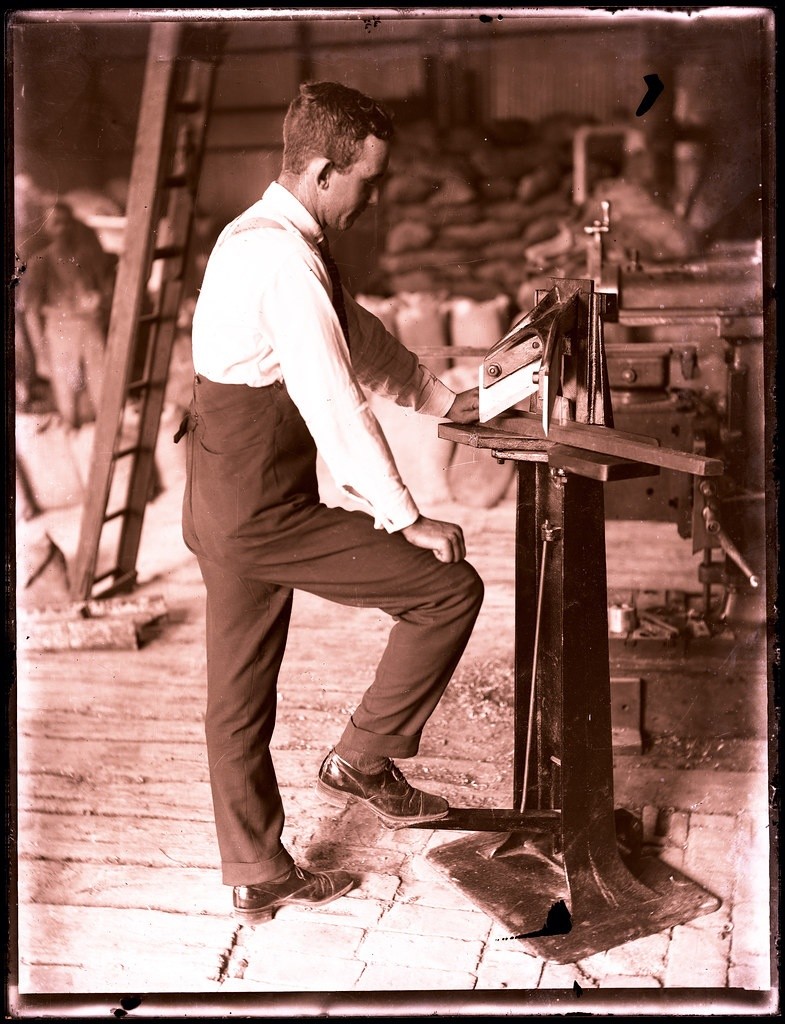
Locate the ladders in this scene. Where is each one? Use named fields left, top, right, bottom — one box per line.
left=66, top=21, right=234, bottom=602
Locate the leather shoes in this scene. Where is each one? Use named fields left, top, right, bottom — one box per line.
left=316, top=746, right=450, bottom=823
left=232, top=864, right=355, bottom=924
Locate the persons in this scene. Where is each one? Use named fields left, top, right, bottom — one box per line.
left=21, top=202, right=114, bottom=437
left=173, top=81, right=511, bottom=925
